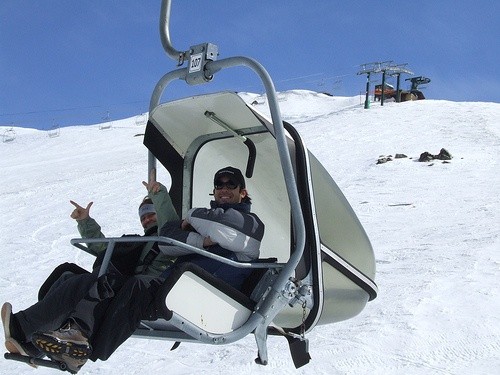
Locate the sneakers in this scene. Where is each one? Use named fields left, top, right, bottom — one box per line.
left=5, top=337, right=45, bottom=361
left=1, top=303, right=25, bottom=344
left=31, top=328, right=94, bottom=359
left=47, top=350, right=88, bottom=375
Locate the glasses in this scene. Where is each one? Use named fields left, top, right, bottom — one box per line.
left=213, top=180, right=240, bottom=190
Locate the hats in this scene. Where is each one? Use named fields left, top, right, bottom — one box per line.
left=214, top=166, right=245, bottom=190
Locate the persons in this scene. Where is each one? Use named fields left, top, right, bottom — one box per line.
left=33, top=167, right=264, bottom=375
left=1, top=169, right=182, bottom=360
